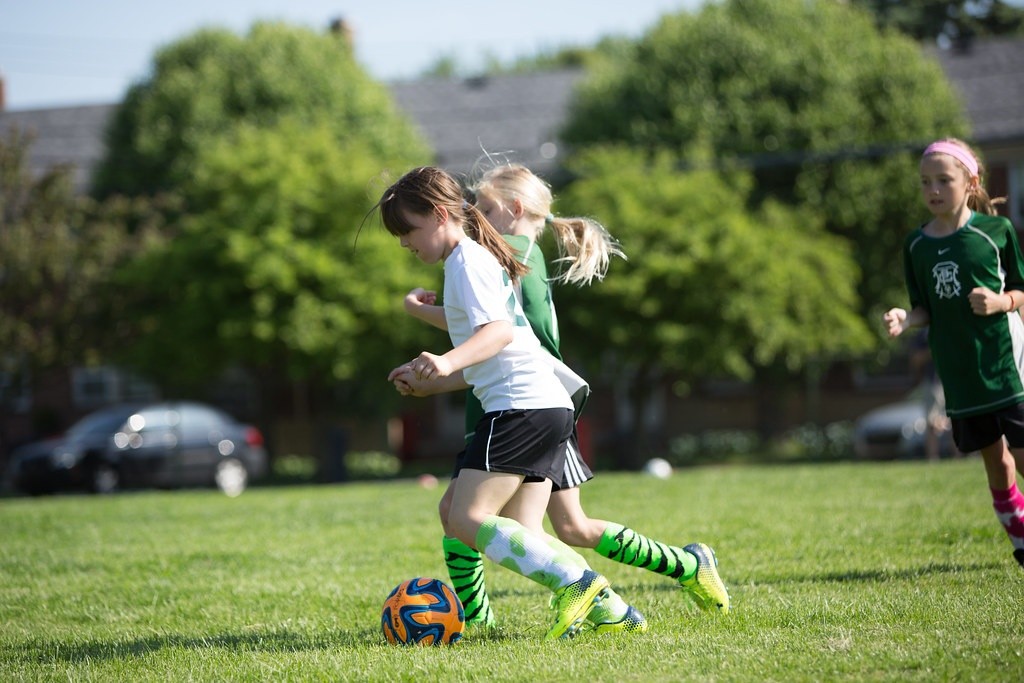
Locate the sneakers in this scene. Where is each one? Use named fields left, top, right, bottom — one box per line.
left=462, top=619, right=499, bottom=638
left=543, top=570, right=610, bottom=640
left=583, top=606, right=647, bottom=641
left=677, top=543, right=732, bottom=619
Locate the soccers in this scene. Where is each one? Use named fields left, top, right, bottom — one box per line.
left=381, top=576, right=466, bottom=647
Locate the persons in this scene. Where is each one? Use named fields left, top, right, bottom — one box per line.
left=882, top=138, right=1024, bottom=569
left=403, top=168, right=731, bottom=637
left=373, top=165, right=611, bottom=642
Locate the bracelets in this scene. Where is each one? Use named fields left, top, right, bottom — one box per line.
left=1004, top=291, right=1015, bottom=312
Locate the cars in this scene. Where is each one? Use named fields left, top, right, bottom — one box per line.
left=0, top=400, right=267, bottom=497
left=850, top=377, right=959, bottom=460
